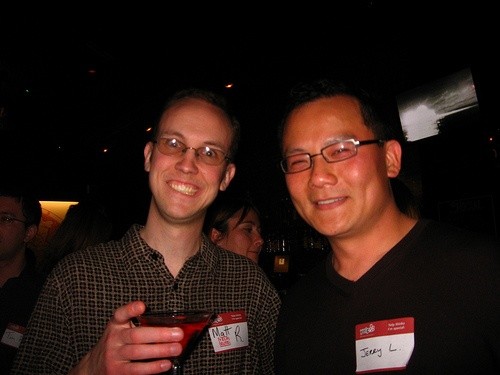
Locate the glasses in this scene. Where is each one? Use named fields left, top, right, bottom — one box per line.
left=155, top=136, right=229, bottom=166
left=0, top=216, right=24, bottom=225
left=281, top=139, right=377, bottom=174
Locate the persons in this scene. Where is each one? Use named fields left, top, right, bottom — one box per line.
left=0, top=177, right=119, bottom=375
left=11, top=88, right=282, bottom=375
left=275, top=74, right=500, bottom=375
left=202, top=195, right=264, bottom=264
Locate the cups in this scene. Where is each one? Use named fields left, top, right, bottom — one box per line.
left=136, top=311, right=215, bottom=375
